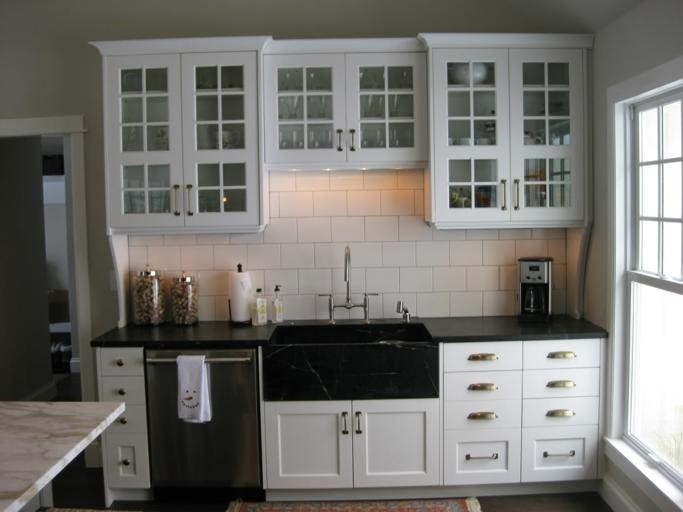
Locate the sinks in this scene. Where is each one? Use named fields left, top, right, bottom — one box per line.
left=266, top=325, right=435, bottom=344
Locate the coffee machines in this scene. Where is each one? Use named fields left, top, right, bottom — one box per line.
left=517, top=256, right=554, bottom=325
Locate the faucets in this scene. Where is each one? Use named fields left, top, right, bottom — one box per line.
left=340, top=246, right=356, bottom=323
left=396, top=300, right=409, bottom=324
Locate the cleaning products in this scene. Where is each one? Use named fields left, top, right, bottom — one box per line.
left=251, top=288, right=267, bottom=327
left=269, top=284, right=285, bottom=324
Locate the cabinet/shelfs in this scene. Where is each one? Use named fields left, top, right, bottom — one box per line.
left=440, top=341, right=521, bottom=500
left=415, top=31, right=595, bottom=233
left=522, top=336, right=607, bottom=497
left=93, top=347, right=151, bottom=509
left=86, top=33, right=273, bottom=235
left=261, top=398, right=440, bottom=504
left=262, top=36, right=428, bottom=175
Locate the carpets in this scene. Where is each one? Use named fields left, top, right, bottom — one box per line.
left=224, top=495, right=481, bottom=512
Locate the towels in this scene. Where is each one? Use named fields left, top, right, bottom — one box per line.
left=175, top=355, right=214, bottom=425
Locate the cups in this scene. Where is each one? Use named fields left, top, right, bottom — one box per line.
left=358, top=66, right=414, bottom=147
left=277, top=67, right=333, bottom=149
left=122, top=177, right=166, bottom=212
left=476, top=138, right=490, bottom=145
left=447, top=137, right=453, bottom=146
left=459, top=137, right=470, bottom=144
left=198, top=195, right=208, bottom=211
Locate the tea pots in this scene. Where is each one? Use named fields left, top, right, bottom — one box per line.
left=523, top=129, right=543, bottom=144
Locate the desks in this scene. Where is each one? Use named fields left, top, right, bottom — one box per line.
left=0, top=400, right=130, bottom=512
